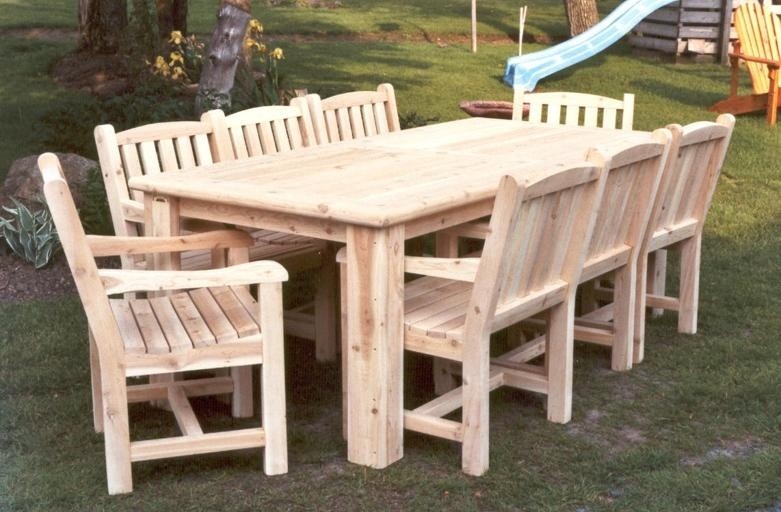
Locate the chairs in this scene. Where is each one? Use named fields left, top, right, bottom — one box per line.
left=531, top=113, right=734, bottom=367
left=335, top=150, right=609, bottom=477
left=94, top=118, right=338, bottom=408
left=432, top=127, right=670, bottom=413
left=436, top=91, right=637, bottom=259
left=204, top=96, right=347, bottom=328
left=37, top=150, right=290, bottom=494
left=710, top=2, right=781, bottom=127
left=304, top=82, right=428, bottom=280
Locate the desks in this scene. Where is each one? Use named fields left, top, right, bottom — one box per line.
left=127, top=116, right=659, bottom=468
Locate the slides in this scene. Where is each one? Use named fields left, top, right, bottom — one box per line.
left=504, top=0, right=676, bottom=93
